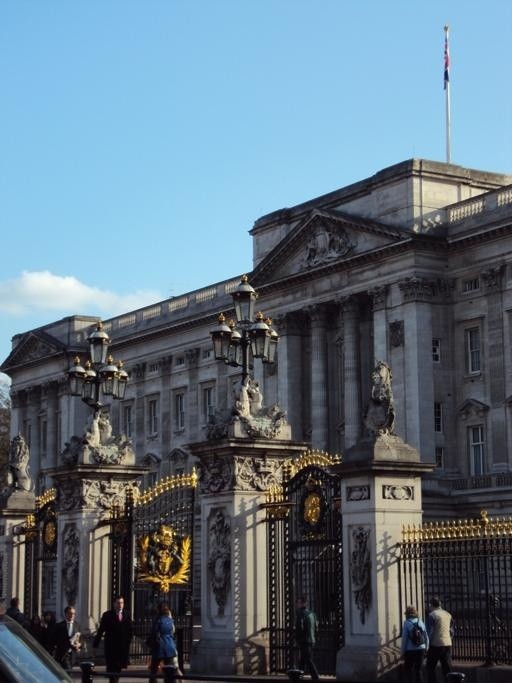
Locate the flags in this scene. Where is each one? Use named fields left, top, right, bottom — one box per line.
left=442, top=35, right=449, bottom=91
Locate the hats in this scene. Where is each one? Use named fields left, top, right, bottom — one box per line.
left=404, top=606, right=418, bottom=617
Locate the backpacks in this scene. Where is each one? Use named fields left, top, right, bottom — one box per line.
left=407, top=620, right=427, bottom=647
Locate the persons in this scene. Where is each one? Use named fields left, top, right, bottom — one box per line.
left=426, top=598, right=454, bottom=682
left=0, top=596, right=184, bottom=683
left=400, top=607, right=430, bottom=682
left=295, top=597, right=320, bottom=683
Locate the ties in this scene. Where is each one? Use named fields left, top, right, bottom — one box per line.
left=118, top=610, right=123, bottom=621
left=67, top=624, right=73, bottom=636
